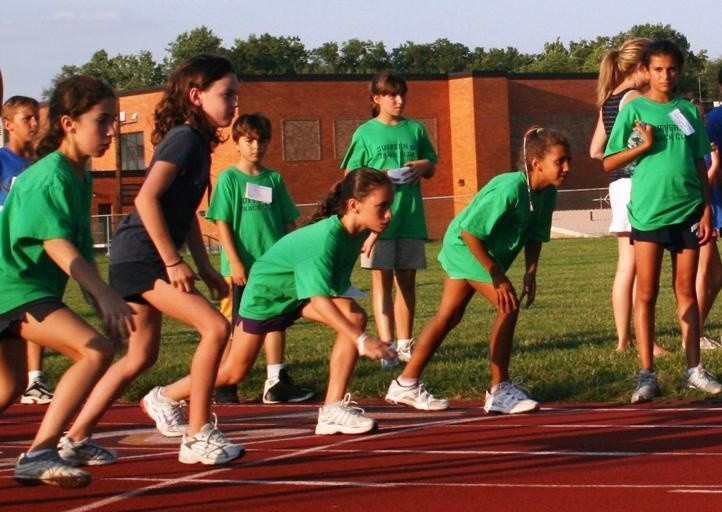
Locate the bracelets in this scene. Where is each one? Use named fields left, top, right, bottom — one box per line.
left=163, top=256, right=185, bottom=268
left=357, top=328, right=371, bottom=358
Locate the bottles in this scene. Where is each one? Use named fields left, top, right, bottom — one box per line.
left=621, top=120, right=646, bottom=175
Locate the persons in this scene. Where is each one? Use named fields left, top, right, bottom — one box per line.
left=3, top=92, right=56, bottom=405
left=204, top=111, right=316, bottom=405
left=138, top=165, right=396, bottom=438
left=598, top=40, right=721, bottom=406
left=678, top=103, right=721, bottom=351
left=337, top=68, right=442, bottom=373
left=3, top=74, right=140, bottom=491
left=587, top=33, right=676, bottom=361
left=54, top=50, right=249, bottom=467
left=382, top=121, right=573, bottom=420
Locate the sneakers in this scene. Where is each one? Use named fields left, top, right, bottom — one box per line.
left=384, top=379, right=449, bottom=411
left=681, top=362, right=721, bottom=395
left=395, top=337, right=416, bottom=362
left=140, top=386, right=188, bottom=438
left=178, top=412, right=241, bottom=466
left=13, top=447, right=91, bottom=488
left=213, top=384, right=240, bottom=405
left=681, top=336, right=720, bottom=350
left=315, top=393, right=378, bottom=435
left=56, top=436, right=117, bottom=467
left=263, top=368, right=315, bottom=404
left=483, top=376, right=539, bottom=414
left=20, top=375, right=54, bottom=405
left=631, top=369, right=662, bottom=403
left=380, top=342, right=400, bottom=370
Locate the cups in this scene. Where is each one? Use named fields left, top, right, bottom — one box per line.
left=387, top=167, right=410, bottom=186
